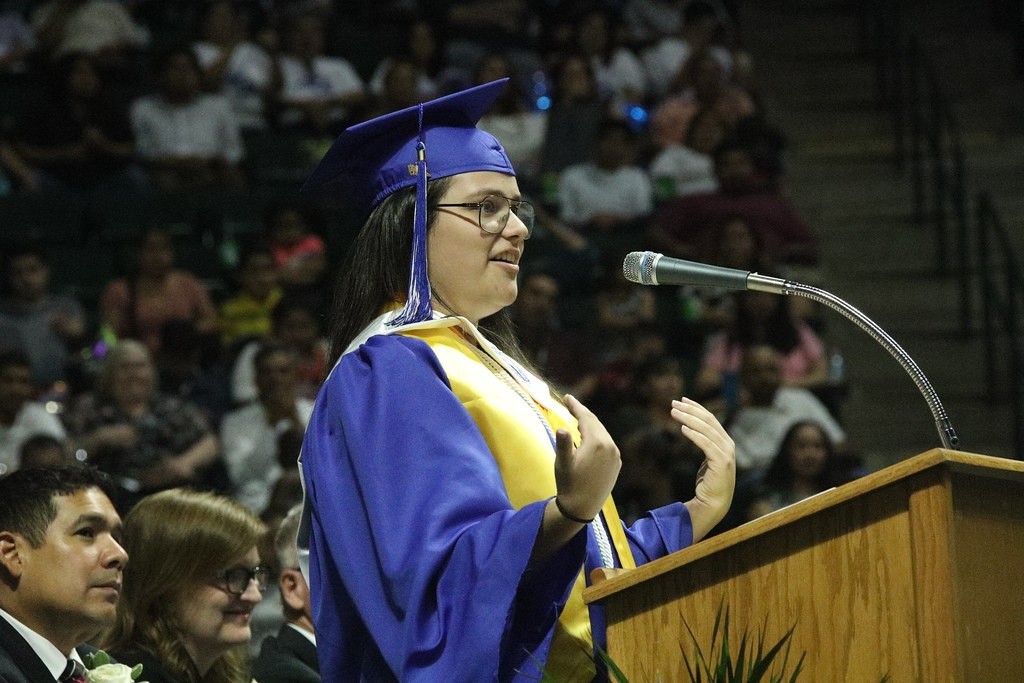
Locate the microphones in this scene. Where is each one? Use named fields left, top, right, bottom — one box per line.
left=623, top=250, right=961, bottom=453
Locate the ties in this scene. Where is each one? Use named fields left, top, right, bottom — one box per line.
left=60, top=659, right=86, bottom=683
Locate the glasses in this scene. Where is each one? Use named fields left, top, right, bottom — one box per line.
left=210, top=563, right=272, bottom=595
left=427, top=195, right=535, bottom=240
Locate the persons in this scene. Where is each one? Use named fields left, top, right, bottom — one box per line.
left=0, top=0, right=858, bottom=682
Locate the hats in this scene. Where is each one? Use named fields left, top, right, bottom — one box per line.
left=301, top=78, right=516, bottom=329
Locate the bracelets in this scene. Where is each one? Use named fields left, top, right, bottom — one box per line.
left=555, top=497, right=594, bottom=524
